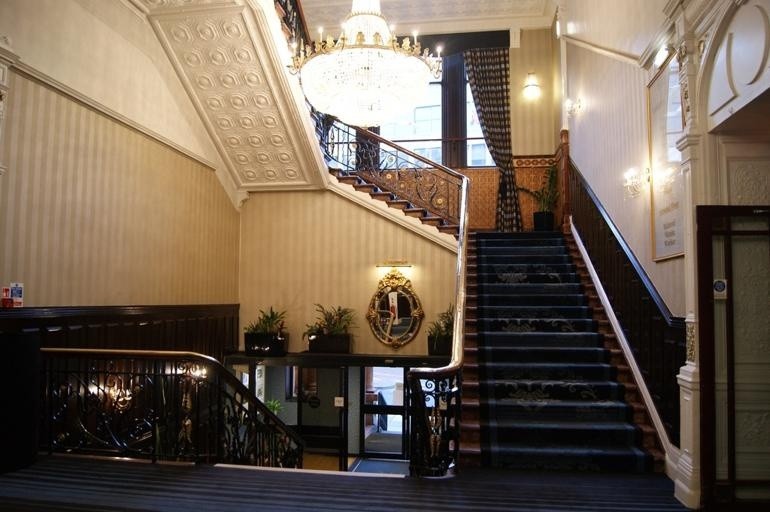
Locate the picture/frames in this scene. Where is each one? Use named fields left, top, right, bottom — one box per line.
left=362, top=267, right=425, bottom=348
left=644, top=45, right=693, bottom=262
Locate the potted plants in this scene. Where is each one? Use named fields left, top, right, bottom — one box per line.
left=299, top=299, right=357, bottom=356
left=513, top=162, right=562, bottom=233
left=237, top=301, right=291, bottom=357
left=427, top=300, right=456, bottom=364
left=264, top=398, right=285, bottom=418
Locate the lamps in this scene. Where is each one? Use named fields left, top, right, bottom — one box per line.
left=369, top=258, right=415, bottom=275
left=284, top=0, right=449, bottom=129
left=520, top=70, right=543, bottom=103
left=620, top=167, right=650, bottom=200
left=563, top=92, right=586, bottom=117
